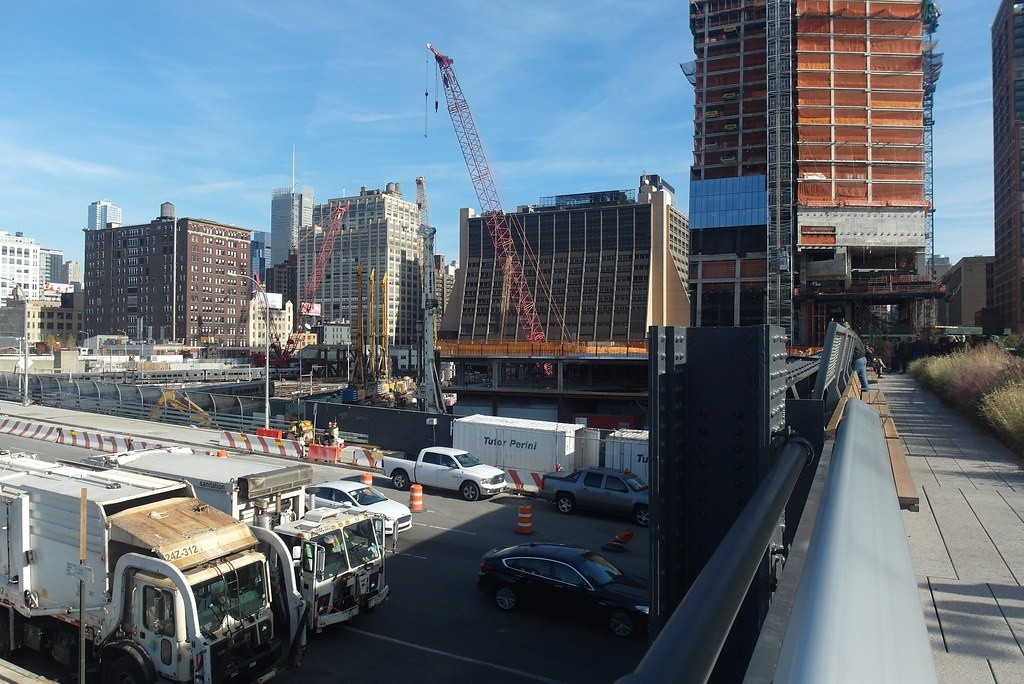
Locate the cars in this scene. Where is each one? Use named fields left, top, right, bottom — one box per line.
left=476, top=539, right=650, bottom=638
left=541, top=463, right=653, bottom=523
left=304, top=476, right=415, bottom=535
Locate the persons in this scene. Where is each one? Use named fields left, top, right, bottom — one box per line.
left=314, top=421, right=339, bottom=446
left=879, top=335, right=998, bottom=374
left=851, top=337, right=868, bottom=392
left=865, top=345, right=891, bottom=379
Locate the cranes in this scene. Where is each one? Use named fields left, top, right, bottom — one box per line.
left=253, top=203, right=349, bottom=368
left=424, top=42, right=572, bottom=376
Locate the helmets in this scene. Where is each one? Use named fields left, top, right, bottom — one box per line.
left=333, top=423, right=338, bottom=428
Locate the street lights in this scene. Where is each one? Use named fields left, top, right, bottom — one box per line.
left=0, top=274, right=30, bottom=398
left=224, top=267, right=274, bottom=431
left=78, top=331, right=89, bottom=348
left=116, top=329, right=126, bottom=357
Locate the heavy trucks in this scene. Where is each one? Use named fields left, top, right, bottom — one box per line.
left=81, top=444, right=391, bottom=630
left=0, top=444, right=283, bottom=684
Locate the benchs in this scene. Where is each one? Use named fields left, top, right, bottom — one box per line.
left=826, top=367, right=919, bottom=512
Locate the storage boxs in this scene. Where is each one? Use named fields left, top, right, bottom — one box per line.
left=605, top=428, right=649, bottom=484
left=452, top=413, right=599, bottom=482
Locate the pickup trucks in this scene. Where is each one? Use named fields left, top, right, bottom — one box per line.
left=381, top=444, right=509, bottom=500
